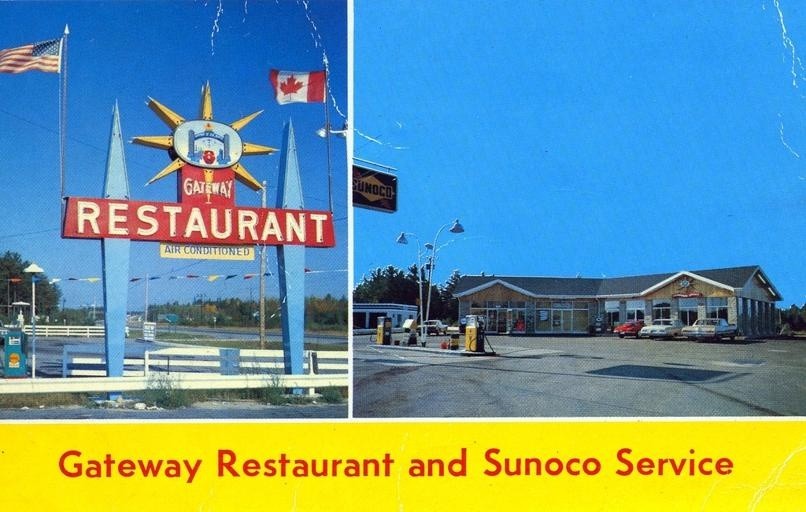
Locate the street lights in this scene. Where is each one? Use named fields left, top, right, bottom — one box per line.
left=394, top=218, right=467, bottom=349
left=22, top=260, right=46, bottom=377
left=11, top=300, right=30, bottom=334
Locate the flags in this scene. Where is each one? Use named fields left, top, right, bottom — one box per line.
left=0, top=36, right=65, bottom=74
left=269, top=68, right=326, bottom=106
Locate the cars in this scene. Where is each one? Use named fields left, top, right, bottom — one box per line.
left=589, top=321, right=616, bottom=337
left=460, top=314, right=483, bottom=335
left=641, top=318, right=685, bottom=339
left=613, top=319, right=648, bottom=338
left=415, top=317, right=447, bottom=335
left=681, top=316, right=738, bottom=340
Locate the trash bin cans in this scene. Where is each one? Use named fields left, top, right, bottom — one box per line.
left=589, top=325, right=596, bottom=337
